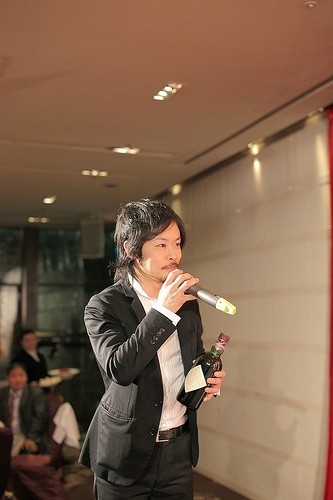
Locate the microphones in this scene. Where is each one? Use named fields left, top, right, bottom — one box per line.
left=173, top=274, right=237, bottom=314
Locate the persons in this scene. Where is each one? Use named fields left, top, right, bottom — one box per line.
left=78, top=199, right=225, bottom=500
left=7, top=328, right=74, bottom=395
left=0, top=364, right=53, bottom=455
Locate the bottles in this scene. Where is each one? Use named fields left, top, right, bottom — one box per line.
left=175, top=332, right=230, bottom=411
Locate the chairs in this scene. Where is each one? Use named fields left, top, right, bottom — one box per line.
left=0, top=428, right=13, bottom=500
left=7, top=394, right=68, bottom=500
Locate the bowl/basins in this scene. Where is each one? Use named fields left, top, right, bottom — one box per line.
left=46, top=367, right=80, bottom=380
